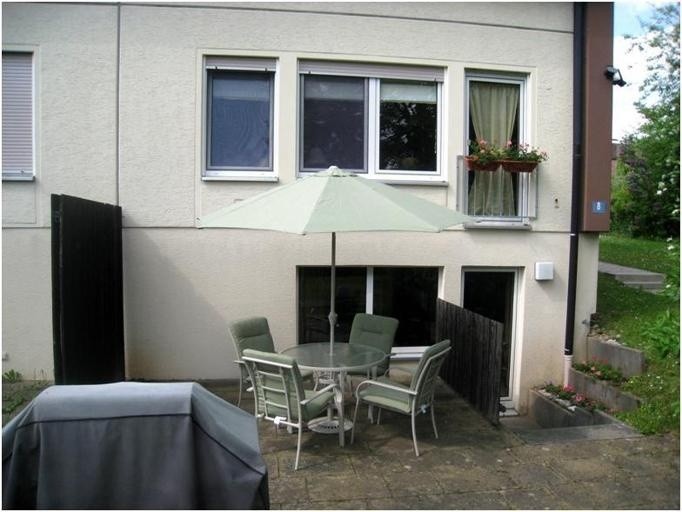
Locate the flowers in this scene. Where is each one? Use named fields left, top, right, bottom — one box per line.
left=467, top=138, right=503, bottom=165
left=502, top=141, right=550, bottom=162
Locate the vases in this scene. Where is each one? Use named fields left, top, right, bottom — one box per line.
left=501, top=159, right=538, bottom=173
left=465, top=156, right=503, bottom=170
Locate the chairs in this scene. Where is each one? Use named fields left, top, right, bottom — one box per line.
left=227, top=313, right=451, bottom=469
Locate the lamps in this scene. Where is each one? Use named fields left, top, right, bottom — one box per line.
left=604, top=65, right=626, bottom=87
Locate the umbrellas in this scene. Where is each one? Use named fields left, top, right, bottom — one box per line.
left=196, top=165, right=476, bottom=419
